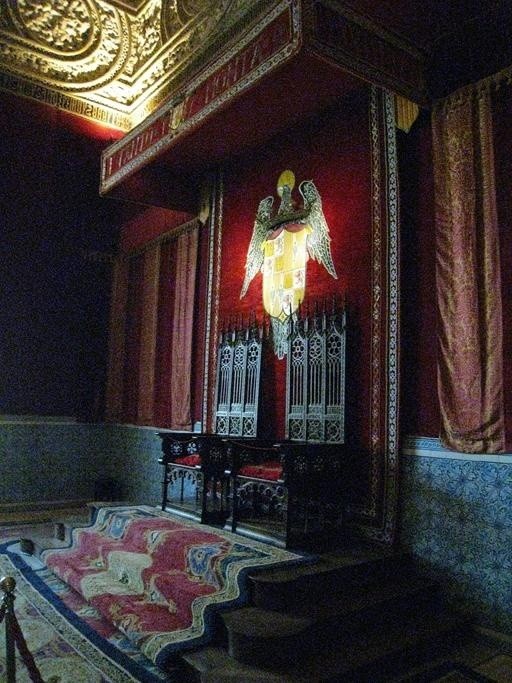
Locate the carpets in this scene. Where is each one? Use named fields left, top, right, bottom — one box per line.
left=0, top=502, right=314, bottom=683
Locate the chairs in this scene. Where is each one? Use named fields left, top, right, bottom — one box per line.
left=155, top=312, right=266, bottom=525
left=219, top=292, right=361, bottom=550
